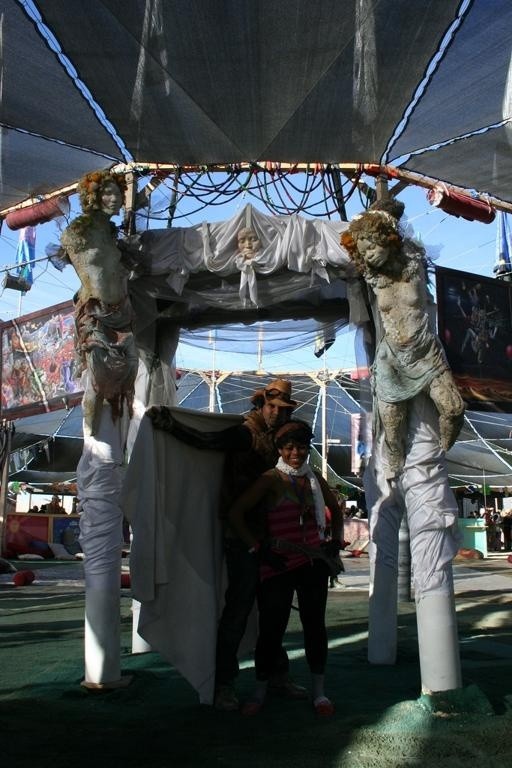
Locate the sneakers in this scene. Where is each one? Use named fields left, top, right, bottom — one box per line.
left=313, top=695, right=334, bottom=716
left=257, top=679, right=308, bottom=698
left=217, top=685, right=264, bottom=717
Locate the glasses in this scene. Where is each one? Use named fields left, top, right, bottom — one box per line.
left=264, top=388, right=290, bottom=403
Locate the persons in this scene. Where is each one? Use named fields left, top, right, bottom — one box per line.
left=211, top=379, right=307, bottom=711
left=341, top=195, right=465, bottom=483
left=27, top=493, right=68, bottom=514
left=62, top=171, right=139, bottom=438
left=236, top=224, right=265, bottom=260
left=229, top=419, right=343, bottom=718
left=458, top=292, right=501, bottom=363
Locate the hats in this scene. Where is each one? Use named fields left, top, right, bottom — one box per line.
left=274, top=422, right=316, bottom=443
left=262, top=379, right=297, bottom=406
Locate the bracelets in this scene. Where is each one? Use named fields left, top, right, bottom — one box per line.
left=248, top=544, right=260, bottom=555
